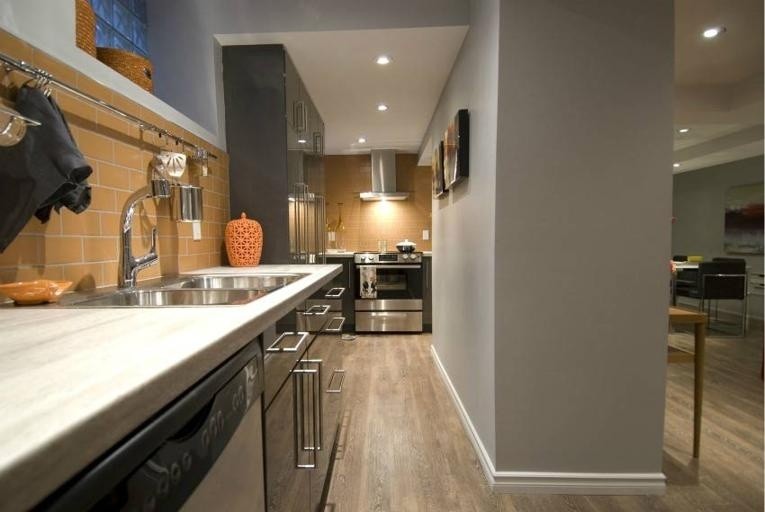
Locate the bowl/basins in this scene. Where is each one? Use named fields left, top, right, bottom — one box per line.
left=396, top=239, right=416, bottom=252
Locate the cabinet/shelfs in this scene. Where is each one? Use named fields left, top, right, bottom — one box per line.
left=221, top=43, right=328, bottom=264
left=301, top=276, right=348, bottom=453
left=255, top=293, right=332, bottom=512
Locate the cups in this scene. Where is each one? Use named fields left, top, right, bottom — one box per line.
left=326, top=248, right=346, bottom=254
left=378, top=240, right=387, bottom=254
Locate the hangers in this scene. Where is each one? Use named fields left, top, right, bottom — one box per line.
left=6, top=55, right=58, bottom=98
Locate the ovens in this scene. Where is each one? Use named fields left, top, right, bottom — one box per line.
left=355, top=266, right=424, bottom=334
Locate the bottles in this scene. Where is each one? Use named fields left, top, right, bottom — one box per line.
left=184, top=145, right=207, bottom=160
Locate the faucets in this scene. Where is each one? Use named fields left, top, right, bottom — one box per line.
left=115, top=179, right=170, bottom=286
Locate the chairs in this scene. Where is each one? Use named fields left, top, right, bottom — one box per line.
left=670, top=249, right=765, bottom=342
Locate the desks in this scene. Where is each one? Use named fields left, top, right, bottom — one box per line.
left=666, top=303, right=711, bottom=460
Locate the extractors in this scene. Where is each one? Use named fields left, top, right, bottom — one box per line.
left=359, top=150, right=410, bottom=201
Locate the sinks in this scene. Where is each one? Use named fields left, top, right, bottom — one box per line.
left=160, top=272, right=311, bottom=291
left=52, top=285, right=273, bottom=307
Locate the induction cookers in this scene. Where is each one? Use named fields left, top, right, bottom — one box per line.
left=355, top=250, right=422, bottom=264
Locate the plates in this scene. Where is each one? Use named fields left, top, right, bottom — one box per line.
left=423, top=252, right=432, bottom=255
left=1, top=279, right=72, bottom=304
